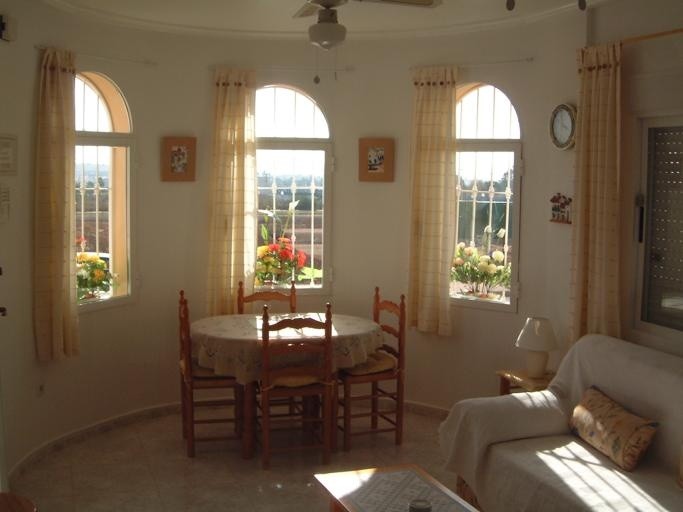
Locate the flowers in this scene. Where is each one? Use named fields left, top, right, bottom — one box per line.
left=75, top=235, right=118, bottom=299
left=255, top=198, right=307, bottom=286
left=451, top=241, right=510, bottom=294
left=254, top=246, right=280, bottom=284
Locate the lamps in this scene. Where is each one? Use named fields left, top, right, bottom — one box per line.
left=307, top=1, right=350, bottom=84
left=516, top=316, right=558, bottom=374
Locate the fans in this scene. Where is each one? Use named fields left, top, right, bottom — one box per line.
left=292, top=1, right=443, bottom=84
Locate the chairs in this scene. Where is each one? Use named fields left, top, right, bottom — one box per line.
left=337, top=286, right=406, bottom=451
left=254, top=304, right=334, bottom=466
left=179, top=290, right=247, bottom=458
left=238, top=279, right=296, bottom=414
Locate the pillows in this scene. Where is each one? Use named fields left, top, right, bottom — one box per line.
left=570, top=387, right=660, bottom=471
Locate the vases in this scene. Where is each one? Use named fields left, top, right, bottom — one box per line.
left=455, top=290, right=500, bottom=300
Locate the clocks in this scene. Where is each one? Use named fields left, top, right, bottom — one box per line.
left=548, top=103, right=577, bottom=149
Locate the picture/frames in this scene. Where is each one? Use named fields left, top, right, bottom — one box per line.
left=358, top=137, right=395, bottom=180
left=161, top=136, right=197, bottom=181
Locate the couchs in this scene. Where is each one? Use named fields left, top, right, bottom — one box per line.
left=439, top=335, right=683, bottom=510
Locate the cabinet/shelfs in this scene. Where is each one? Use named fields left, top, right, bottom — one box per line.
left=497, top=370, right=553, bottom=395
